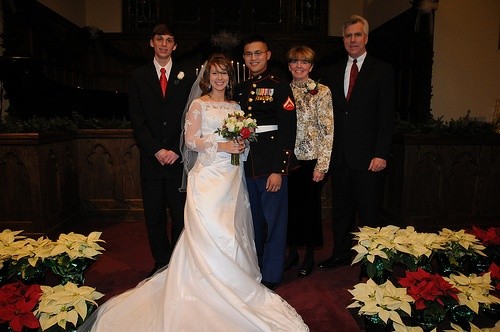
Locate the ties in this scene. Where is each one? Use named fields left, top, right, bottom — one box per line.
left=345, top=59, right=359, bottom=105
left=159, top=68, right=168, bottom=98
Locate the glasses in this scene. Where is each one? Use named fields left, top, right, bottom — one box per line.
left=244, top=51, right=267, bottom=57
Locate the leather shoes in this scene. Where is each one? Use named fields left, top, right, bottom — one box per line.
left=317, top=255, right=352, bottom=271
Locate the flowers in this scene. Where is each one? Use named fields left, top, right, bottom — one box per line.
left=173, top=71, right=185, bottom=85
left=393, top=225, right=438, bottom=273
left=434, top=228, right=488, bottom=275
left=442, top=271, right=500, bottom=328
left=443, top=321, right=500, bottom=332
left=397, top=267, right=464, bottom=330
left=214, top=111, right=258, bottom=166
left=345, top=276, right=416, bottom=332
left=479, top=263, right=500, bottom=290
left=465, top=227, right=500, bottom=266
left=33, top=231, right=106, bottom=286
left=349, top=225, right=400, bottom=285
left=0, top=229, right=46, bottom=285
left=0, top=281, right=43, bottom=332
left=304, top=79, right=321, bottom=95
left=392, top=322, right=437, bottom=332
left=33, top=281, right=105, bottom=332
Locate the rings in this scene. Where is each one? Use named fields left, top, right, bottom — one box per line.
left=321, top=178, right=323, bottom=180
left=279, top=187, right=280, bottom=189
left=235, top=150, right=236, bottom=151
left=276, top=187, right=278, bottom=189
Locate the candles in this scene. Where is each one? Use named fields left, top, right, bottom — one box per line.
left=243, top=64, right=246, bottom=81
left=237, top=62, right=240, bottom=83
left=196, top=68, right=198, bottom=76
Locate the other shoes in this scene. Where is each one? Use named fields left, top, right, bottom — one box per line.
left=297, top=259, right=314, bottom=278
left=283, top=254, right=301, bottom=264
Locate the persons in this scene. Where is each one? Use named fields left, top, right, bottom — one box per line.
left=283, top=45, right=334, bottom=279
left=233, top=38, right=297, bottom=290
left=316, top=15, right=394, bottom=281
left=131, top=24, right=196, bottom=280
left=76, top=53, right=310, bottom=332
